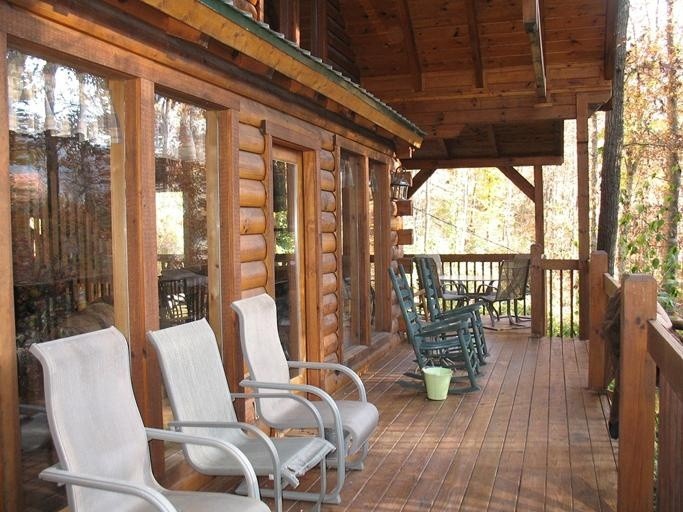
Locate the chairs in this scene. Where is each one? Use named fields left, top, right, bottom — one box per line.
left=387, top=252, right=532, bottom=394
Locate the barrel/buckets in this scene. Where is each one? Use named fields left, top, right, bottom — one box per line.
left=421, top=366, right=455, bottom=402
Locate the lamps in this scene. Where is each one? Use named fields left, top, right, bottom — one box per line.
left=389, top=166, right=412, bottom=203
